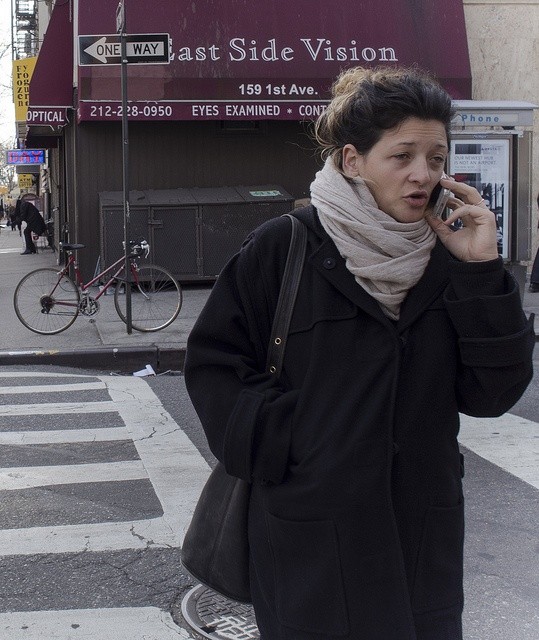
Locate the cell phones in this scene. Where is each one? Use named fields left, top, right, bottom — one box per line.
left=428, top=169, right=451, bottom=220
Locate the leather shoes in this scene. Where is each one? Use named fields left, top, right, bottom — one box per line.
left=30, top=248, right=36, bottom=253
left=20, top=249, right=32, bottom=255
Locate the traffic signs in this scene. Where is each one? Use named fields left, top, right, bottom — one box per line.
left=76, top=33, right=171, bottom=67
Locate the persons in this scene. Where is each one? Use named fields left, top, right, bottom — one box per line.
left=7, top=199, right=46, bottom=255
left=184, top=66, right=533, bottom=640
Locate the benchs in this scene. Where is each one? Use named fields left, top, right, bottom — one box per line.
left=33, top=219, right=54, bottom=254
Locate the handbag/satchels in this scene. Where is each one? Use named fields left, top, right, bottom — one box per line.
left=181, top=459, right=252, bottom=605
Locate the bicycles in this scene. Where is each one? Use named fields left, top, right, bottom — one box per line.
left=13, top=237, right=183, bottom=332
